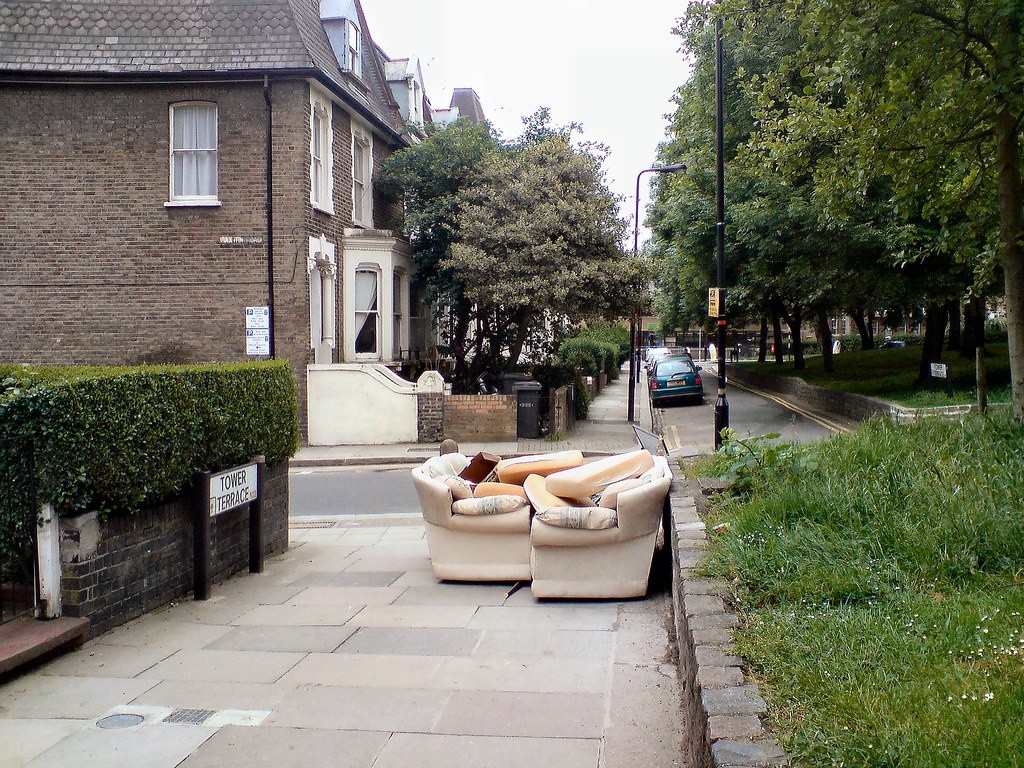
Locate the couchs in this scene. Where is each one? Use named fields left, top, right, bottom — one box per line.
left=530, top=454, right=673, bottom=598
left=411, top=453, right=532, bottom=582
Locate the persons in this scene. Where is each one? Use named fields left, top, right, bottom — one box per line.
left=682, top=349, right=692, bottom=361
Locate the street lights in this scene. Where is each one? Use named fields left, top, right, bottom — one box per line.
left=628, top=164, right=698, bottom=425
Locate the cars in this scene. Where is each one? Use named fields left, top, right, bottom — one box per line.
left=879, top=342, right=906, bottom=349
left=649, top=356, right=704, bottom=409
left=644, top=346, right=675, bottom=378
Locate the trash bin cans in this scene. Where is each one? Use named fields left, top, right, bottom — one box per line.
left=512, top=382, right=542, bottom=439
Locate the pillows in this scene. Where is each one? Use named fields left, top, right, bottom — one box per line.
left=534, top=506, right=616, bottom=531
left=435, top=474, right=473, bottom=498
left=452, top=494, right=526, bottom=515
